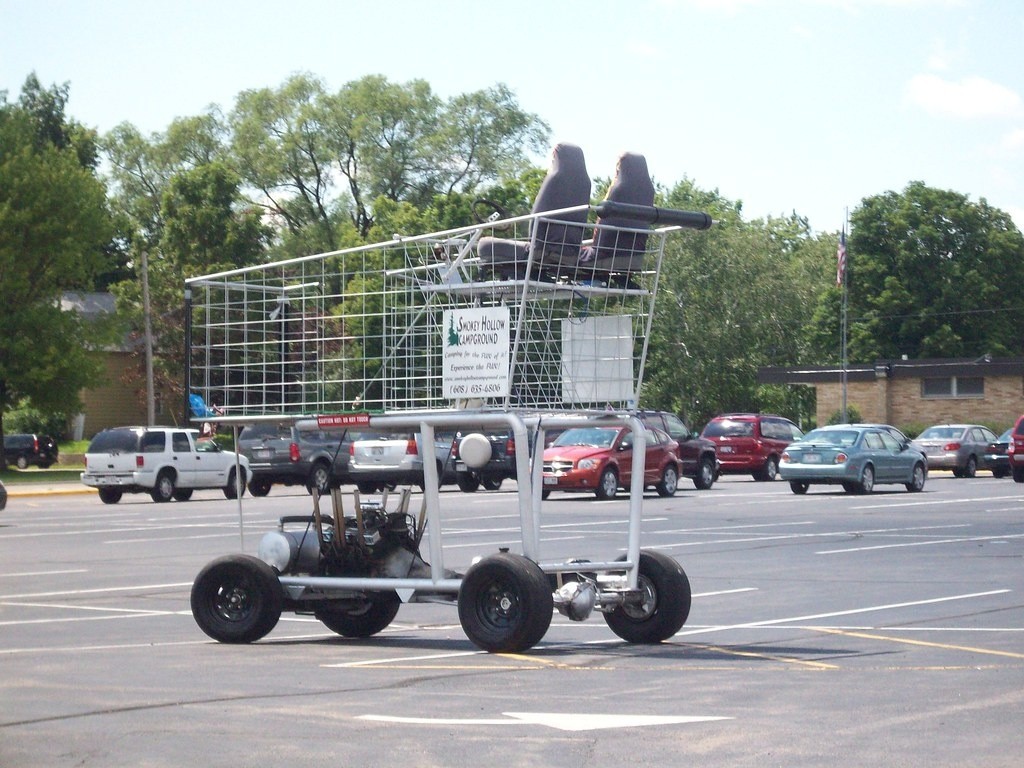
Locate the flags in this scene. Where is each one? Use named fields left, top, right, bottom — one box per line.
left=836, top=222, right=846, bottom=287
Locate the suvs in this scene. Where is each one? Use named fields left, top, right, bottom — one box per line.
left=238, top=424, right=375, bottom=498
left=450, top=429, right=565, bottom=493
left=3, top=432, right=58, bottom=470
left=347, top=432, right=458, bottom=494
left=1007, top=415, right=1024, bottom=483
left=80, top=425, right=254, bottom=505
left=631, top=408, right=721, bottom=491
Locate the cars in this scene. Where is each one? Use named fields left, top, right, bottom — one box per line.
left=823, top=423, right=927, bottom=459
left=982, top=427, right=1015, bottom=478
left=779, top=426, right=929, bottom=495
left=541, top=426, right=682, bottom=501
left=912, top=423, right=999, bottom=478
left=699, top=412, right=806, bottom=482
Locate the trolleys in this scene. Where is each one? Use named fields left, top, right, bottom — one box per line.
left=185, top=142, right=712, bottom=654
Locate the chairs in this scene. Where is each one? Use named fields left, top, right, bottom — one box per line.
left=475, top=144, right=590, bottom=281
left=580, top=150, right=655, bottom=287
